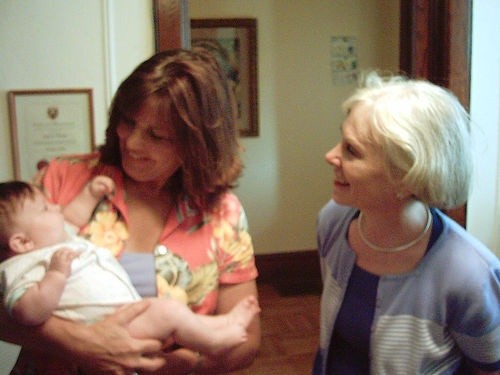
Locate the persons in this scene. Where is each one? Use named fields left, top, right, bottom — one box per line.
left=0, top=181, right=261, bottom=359
left=1, top=49, right=263, bottom=375
left=312, top=75, right=500, bottom=375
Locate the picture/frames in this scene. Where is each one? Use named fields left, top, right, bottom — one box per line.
left=190, top=16, right=259, bottom=139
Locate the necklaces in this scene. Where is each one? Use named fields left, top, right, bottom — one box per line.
left=358, top=207, right=432, bottom=253
